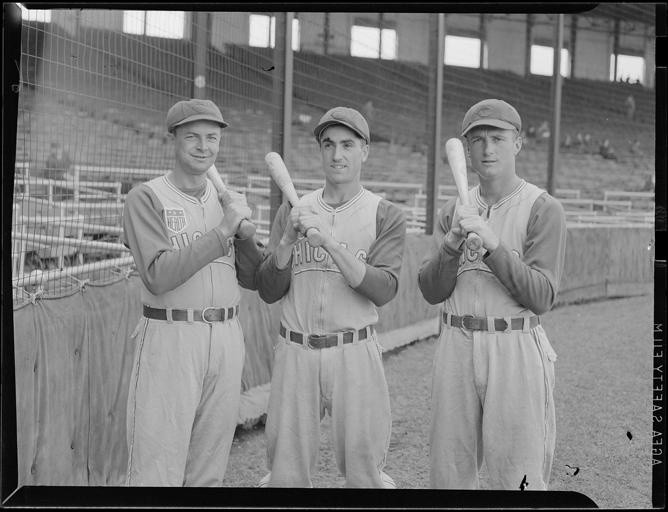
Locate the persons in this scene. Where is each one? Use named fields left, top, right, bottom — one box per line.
left=357, top=100, right=376, bottom=121
left=43, top=142, right=76, bottom=204
left=122, top=98, right=264, bottom=488
left=416, top=97, right=567, bottom=491
left=517, top=121, right=650, bottom=163
left=644, top=174, right=655, bottom=192
left=257, top=106, right=407, bottom=488
left=625, top=94, right=636, bottom=121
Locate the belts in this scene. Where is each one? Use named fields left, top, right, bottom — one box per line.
left=442, top=312, right=541, bottom=331
left=143, top=303, right=240, bottom=323
left=281, top=321, right=375, bottom=349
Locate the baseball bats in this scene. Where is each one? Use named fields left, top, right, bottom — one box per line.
left=264, top=153, right=323, bottom=247
left=204, top=163, right=255, bottom=241
left=446, top=138, right=483, bottom=251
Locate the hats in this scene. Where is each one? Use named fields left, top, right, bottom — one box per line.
left=314, top=106, right=369, bottom=144
left=166, top=99, right=227, bottom=133
left=461, top=99, right=521, bottom=138
